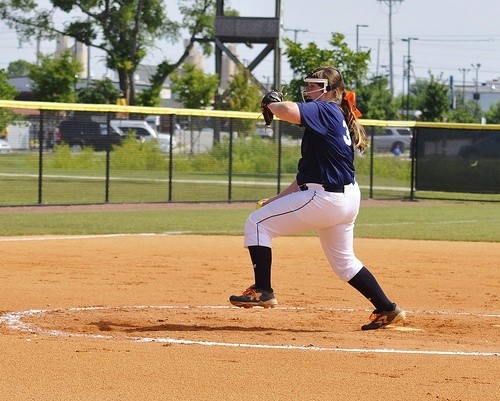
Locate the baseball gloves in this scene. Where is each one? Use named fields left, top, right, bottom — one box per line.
left=261, top=90, right=283, bottom=125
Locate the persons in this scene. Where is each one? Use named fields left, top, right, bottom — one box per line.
left=227, top=68, right=405, bottom=333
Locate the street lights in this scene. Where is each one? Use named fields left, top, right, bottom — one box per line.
left=401, top=37, right=419, bottom=121
left=458, top=68, right=471, bottom=106
left=355, top=24, right=369, bottom=53
left=471, top=63, right=482, bottom=112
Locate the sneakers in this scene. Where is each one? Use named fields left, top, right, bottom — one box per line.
left=229, top=284, right=278, bottom=309
left=362, top=303, right=406, bottom=331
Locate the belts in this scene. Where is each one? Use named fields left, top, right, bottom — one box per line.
left=300, top=183, right=344, bottom=193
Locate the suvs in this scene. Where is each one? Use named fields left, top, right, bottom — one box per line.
left=110, top=120, right=160, bottom=147
left=56, top=120, right=141, bottom=151
left=359, top=127, right=413, bottom=153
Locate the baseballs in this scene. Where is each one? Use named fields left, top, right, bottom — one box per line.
left=256, top=202, right=263, bottom=209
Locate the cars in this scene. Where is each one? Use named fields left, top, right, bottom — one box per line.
left=255, top=121, right=273, bottom=138
